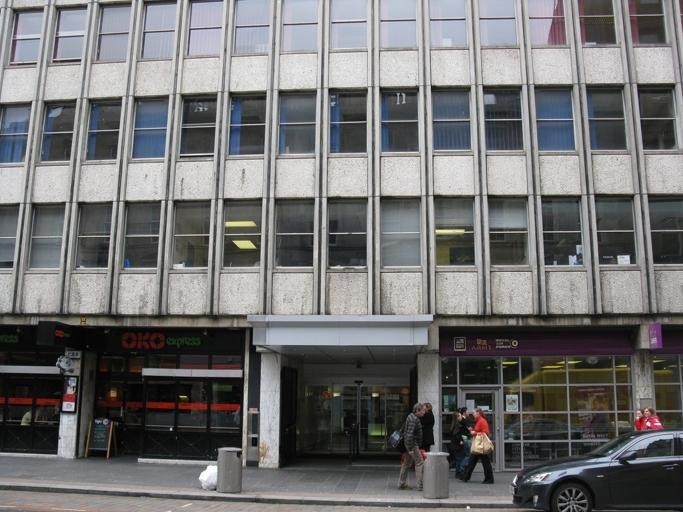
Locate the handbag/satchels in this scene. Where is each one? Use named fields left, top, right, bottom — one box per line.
left=469, top=431, right=495, bottom=456
left=399, top=448, right=427, bottom=467
left=387, top=428, right=405, bottom=451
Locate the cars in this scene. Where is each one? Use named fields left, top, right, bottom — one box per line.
left=509, top=429, right=682, bottom=512
left=503, top=418, right=582, bottom=455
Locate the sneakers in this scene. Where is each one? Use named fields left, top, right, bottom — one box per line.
left=397, top=474, right=494, bottom=490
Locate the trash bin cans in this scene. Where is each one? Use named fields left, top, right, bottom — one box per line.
left=423, top=452, right=450, bottom=499
left=217, top=447, right=242, bottom=493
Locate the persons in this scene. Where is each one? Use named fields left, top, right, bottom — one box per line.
left=458, top=406, right=494, bottom=484
left=591, top=399, right=608, bottom=434
left=635, top=407, right=663, bottom=430
left=388, top=402, right=424, bottom=490
left=20, top=404, right=43, bottom=425
left=632, top=408, right=643, bottom=430
left=419, top=402, right=434, bottom=452
left=454, top=407, right=472, bottom=479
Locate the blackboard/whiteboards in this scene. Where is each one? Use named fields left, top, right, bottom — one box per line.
left=85, top=419, right=112, bottom=449
